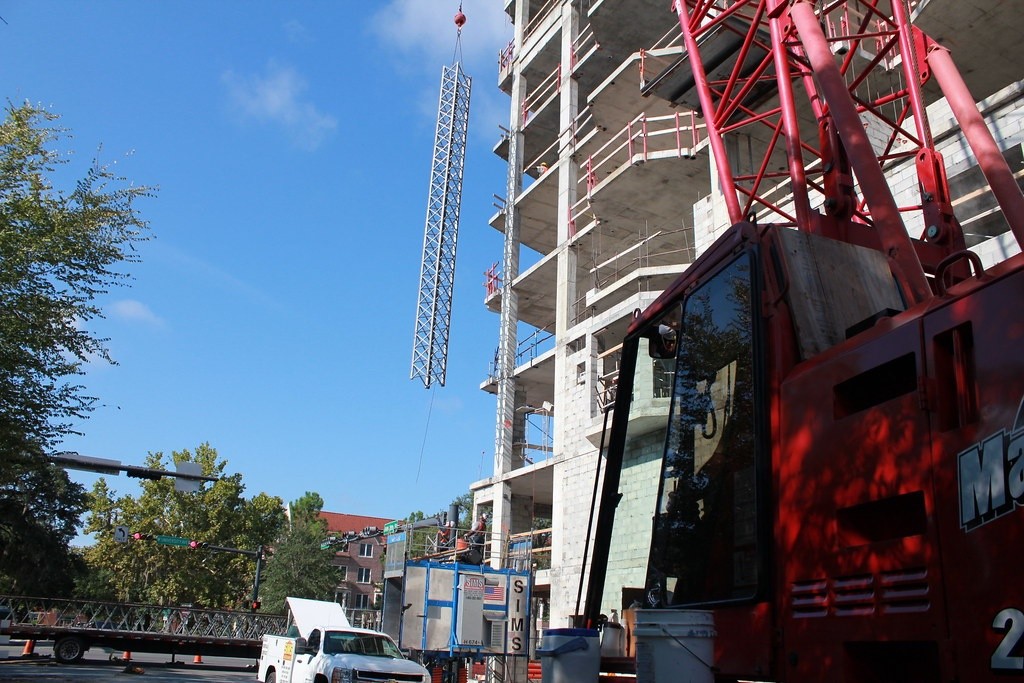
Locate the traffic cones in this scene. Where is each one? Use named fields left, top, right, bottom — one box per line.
left=124, top=665, right=145, bottom=674
left=121, top=652, right=134, bottom=660
left=193, top=655, right=204, bottom=664
left=24, top=639, right=33, bottom=655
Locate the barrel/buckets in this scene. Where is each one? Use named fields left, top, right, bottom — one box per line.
left=536, top=626, right=600, bottom=682
left=632, top=606, right=718, bottom=683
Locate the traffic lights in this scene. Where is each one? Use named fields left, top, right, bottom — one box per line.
left=361, top=526, right=379, bottom=534
left=134, top=531, right=154, bottom=540
left=321, top=535, right=338, bottom=546
left=190, top=540, right=210, bottom=549
left=252, top=601, right=261, bottom=609
left=341, top=531, right=358, bottom=539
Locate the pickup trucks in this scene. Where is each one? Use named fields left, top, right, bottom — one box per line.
left=255, top=596, right=431, bottom=683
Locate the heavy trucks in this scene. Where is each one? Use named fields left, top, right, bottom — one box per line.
left=0, top=592, right=290, bottom=662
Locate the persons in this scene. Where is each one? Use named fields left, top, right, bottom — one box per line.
left=463, top=514, right=488, bottom=553
left=437, top=520, right=456, bottom=552
left=133, top=611, right=154, bottom=632
left=541, top=533, right=551, bottom=547
left=536, top=161, right=548, bottom=176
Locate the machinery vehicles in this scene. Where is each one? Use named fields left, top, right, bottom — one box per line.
left=452, top=0, right=1024, bottom=683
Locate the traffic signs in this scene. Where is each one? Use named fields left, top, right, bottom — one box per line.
left=114, top=525, right=128, bottom=542
